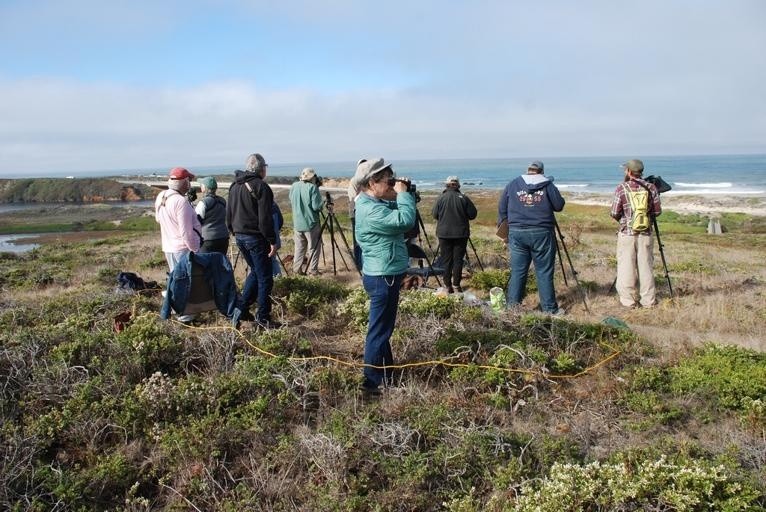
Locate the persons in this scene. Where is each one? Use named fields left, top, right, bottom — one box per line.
left=289, top=168, right=323, bottom=277
left=497, top=161, right=565, bottom=316
left=354, top=157, right=417, bottom=400
left=225, top=154, right=282, bottom=329
left=271, top=201, right=283, bottom=278
left=609, top=160, right=662, bottom=309
left=195, top=176, right=229, bottom=256
left=155, top=168, right=203, bottom=273
left=348, top=159, right=367, bottom=271
left=431, top=176, right=477, bottom=294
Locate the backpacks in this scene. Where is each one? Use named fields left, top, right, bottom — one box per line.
left=619, top=181, right=652, bottom=233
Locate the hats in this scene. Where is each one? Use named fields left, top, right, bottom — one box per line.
left=626, top=159, right=644, bottom=176
left=299, top=168, right=317, bottom=182
left=353, top=157, right=394, bottom=189
left=528, top=160, right=544, bottom=174
left=168, top=166, right=196, bottom=181
left=196, top=175, right=218, bottom=191
left=444, top=175, right=460, bottom=185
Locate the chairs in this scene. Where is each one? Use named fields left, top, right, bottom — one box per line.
left=403, top=244, right=446, bottom=288
left=160, top=251, right=227, bottom=326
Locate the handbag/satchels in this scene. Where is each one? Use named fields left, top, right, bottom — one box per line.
left=193, top=227, right=204, bottom=248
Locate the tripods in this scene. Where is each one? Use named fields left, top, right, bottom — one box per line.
left=233, top=242, right=289, bottom=276
left=432, top=230, right=484, bottom=278
left=504, top=218, right=582, bottom=289
left=303, top=211, right=359, bottom=277
left=320, top=210, right=350, bottom=272
left=608, top=219, right=673, bottom=299
left=413, top=207, right=436, bottom=268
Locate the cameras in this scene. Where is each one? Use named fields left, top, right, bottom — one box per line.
left=391, top=178, right=417, bottom=193
left=188, top=188, right=197, bottom=201
left=545, top=175, right=554, bottom=182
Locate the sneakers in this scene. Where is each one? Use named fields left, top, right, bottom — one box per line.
left=255, top=313, right=283, bottom=329
left=358, top=375, right=396, bottom=399
left=294, top=271, right=323, bottom=277
left=554, top=308, right=566, bottom=316
left=444, top=284, right=464, bottom=294
left=177, top=314, right=196, bottom=325
left=237, top=310, right=255, bottom=323
